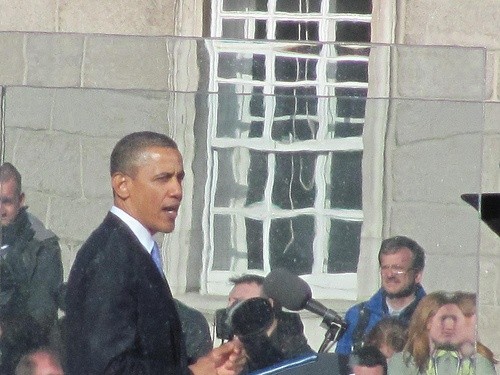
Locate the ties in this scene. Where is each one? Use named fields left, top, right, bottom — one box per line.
left=151, top=241, right=164, bottom=280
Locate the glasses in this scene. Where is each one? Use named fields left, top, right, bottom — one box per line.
left=377, top=263, right=415, bottom=275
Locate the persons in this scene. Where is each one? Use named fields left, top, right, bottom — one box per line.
left=228, top=274, right=316, bottom=374
left=347, top=291, right=500, bottom=375
left=335, top=236, right=426, bottom=355
left=59, top=131, right=247, bottom=375
left=0, top=163, right=63, bottom=375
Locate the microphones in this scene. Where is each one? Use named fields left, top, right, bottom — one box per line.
left=264, top=266, right=348, bottom=328
